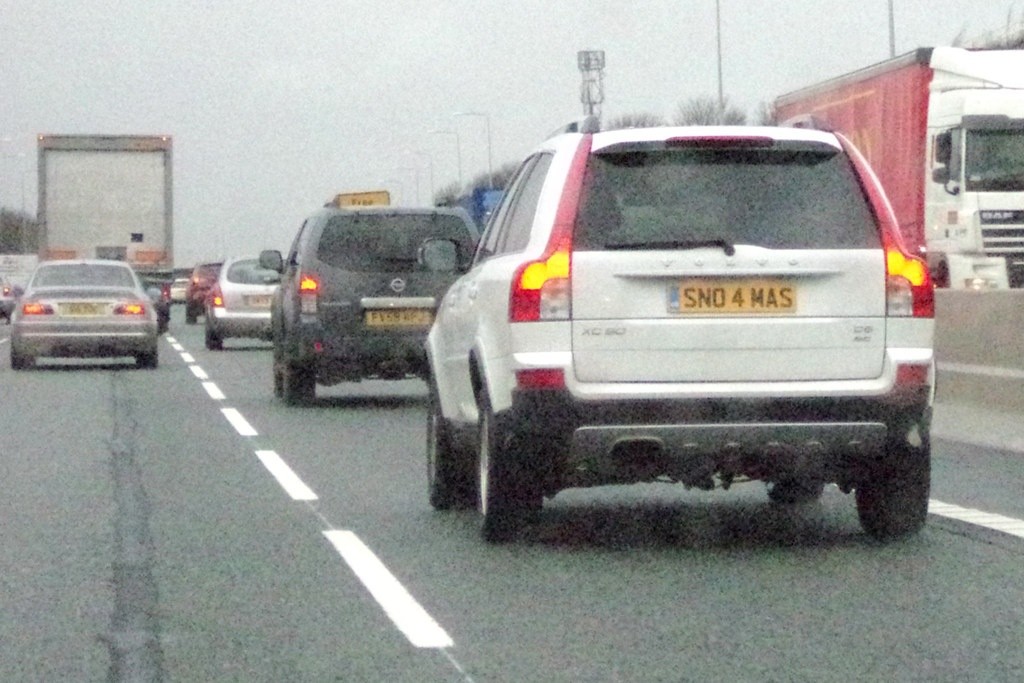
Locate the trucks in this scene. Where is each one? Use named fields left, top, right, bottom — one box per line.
left=35, top=133, right=176, bottom=335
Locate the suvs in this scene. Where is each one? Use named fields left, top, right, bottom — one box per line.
left=418, top=112, right=936, bottom=541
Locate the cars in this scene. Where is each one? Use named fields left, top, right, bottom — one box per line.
left=1, top=254, right=38, bottom=319
left=170, top=265, right=193, bottom=304
left=205, top=251, right=286, bottom=350
left=186, top=261, right=223, bottom=326
left=11, top=260, right=160, bottom=370
left=269, top=189, right=484, bottom=408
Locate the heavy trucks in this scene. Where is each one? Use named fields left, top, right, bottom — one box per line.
left=436, top=186, right=504, bottom=234
left=771, top=42, right=1024, bottom=295
